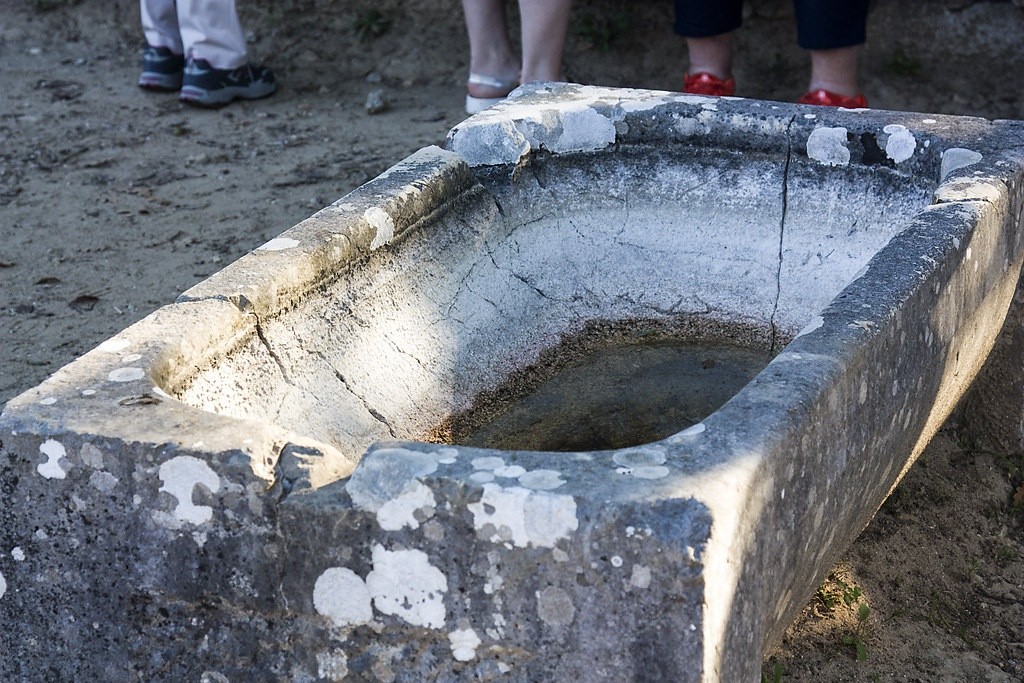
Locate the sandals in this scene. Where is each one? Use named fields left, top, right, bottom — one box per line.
left=465, top=72, right=518, bottom=114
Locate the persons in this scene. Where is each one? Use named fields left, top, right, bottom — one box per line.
left=674, top=0, right=868, bottom=109
left=139, top=0, right=277, bottom=104
left=461, top=0, right=572, bottom=116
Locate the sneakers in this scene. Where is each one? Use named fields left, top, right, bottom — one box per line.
left=179, top=57, right=277, bottom=103
left=137, top=46, right=184, bottom=88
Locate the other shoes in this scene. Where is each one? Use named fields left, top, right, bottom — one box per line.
left=798, top=89, right=867, bottom=110
left=682, top=71, right=735, bottom=97
